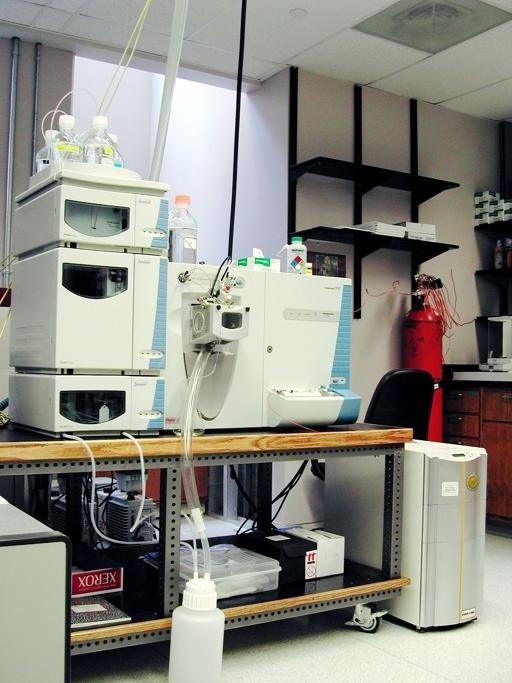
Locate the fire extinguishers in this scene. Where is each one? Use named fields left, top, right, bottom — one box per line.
left=404, top=276, right=446, bottom=442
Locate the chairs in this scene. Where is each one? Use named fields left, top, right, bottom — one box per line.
left=310, top=369, right=435, bottom=481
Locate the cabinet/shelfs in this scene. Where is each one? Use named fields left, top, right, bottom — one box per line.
left=474, top=220, right=512, bottom=320
left=0, top=423, right=411, bottom=656
left=479, top=387, right=512, bottom=519
left=289, top=156, right=460, bottom=266
left=442, top=390, right=480, bottom=447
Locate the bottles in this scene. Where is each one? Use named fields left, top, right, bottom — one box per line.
left=35, top=113, right=126, bottom=173
left=170, top=195, right=198, bottom=264
left=306, top=262, right=312, bottom=275
left=492, top=240, right=504, bottom=268
left=285, top=237, right=308, bottom=273
left=166, top=574, right=228, bottom=682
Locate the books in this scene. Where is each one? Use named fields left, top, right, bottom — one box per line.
left=70, top=596, right=132, bottom=628
left=354, top=219, right=437, bottom=241
